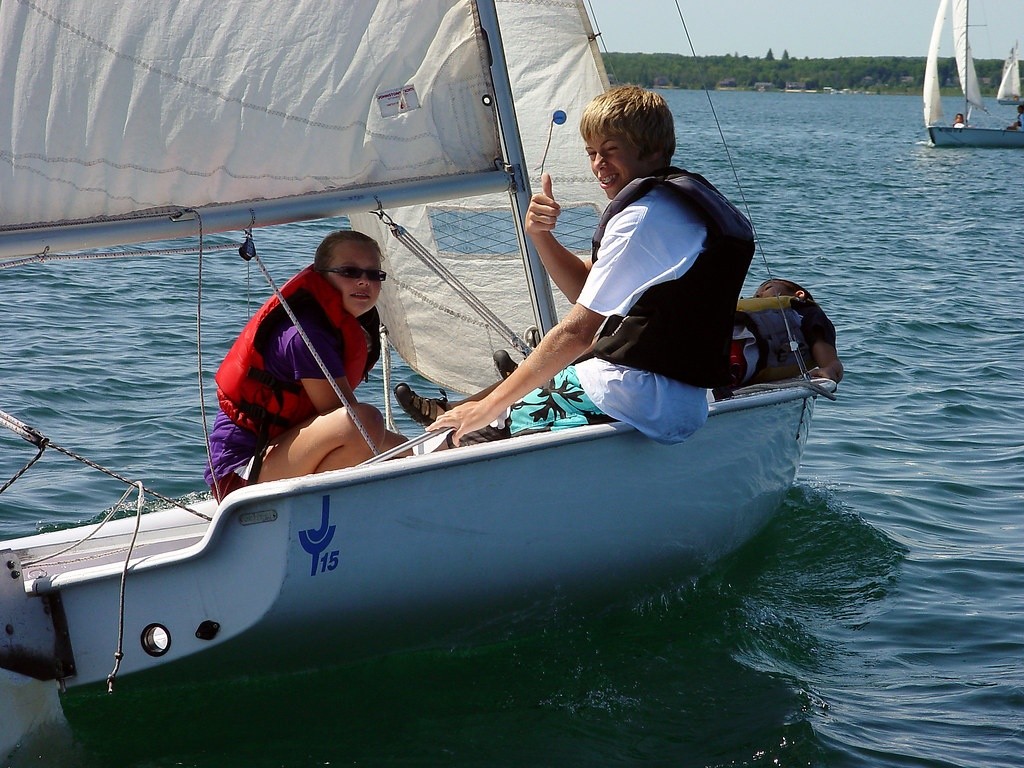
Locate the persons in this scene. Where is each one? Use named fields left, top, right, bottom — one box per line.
left=1006, top=97, right=1024, bottom=132
left=952, top=113, right=963, bottom=128
left=396, top=86, right=844, bottom=448
left=206, top=230, right=412, bottom=503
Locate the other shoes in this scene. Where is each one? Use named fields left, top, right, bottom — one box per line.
left=493, top=350, right=518, bottom=380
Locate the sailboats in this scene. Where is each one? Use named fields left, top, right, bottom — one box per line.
left=922, top=0, right=1024, bottom=147
left=0, top=1, right=837, bottom=689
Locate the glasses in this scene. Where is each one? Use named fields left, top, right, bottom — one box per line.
left=320, top=266, right=386, bottom=281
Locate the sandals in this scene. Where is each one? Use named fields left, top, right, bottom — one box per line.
left=393, top=382, right=453, bottom=428
left=447, top=424, right=511, bottom=449
